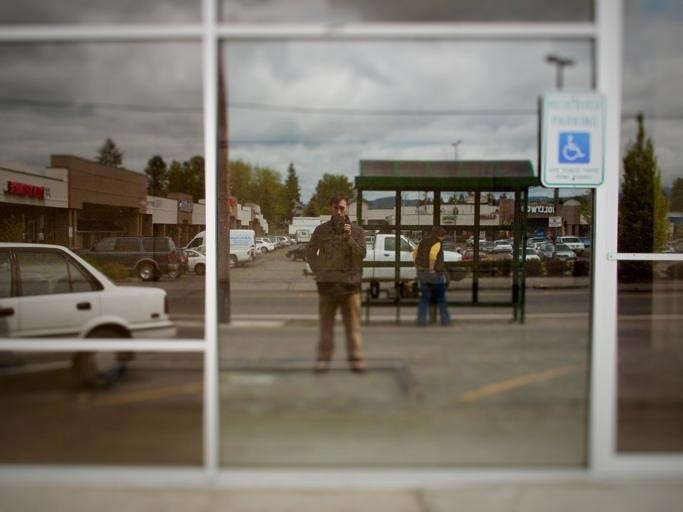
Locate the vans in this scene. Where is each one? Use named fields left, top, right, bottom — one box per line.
left=75, top=235, right=189, bottom=282
left=182, top=229, right=256, bottom=268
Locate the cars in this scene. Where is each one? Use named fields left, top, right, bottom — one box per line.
left=365, top=234, right=592, bottom=265
left=286, top=244, right=309, bottom=261
left=659, top=239, right=683, bottom=253
left=255, top=235, right=297, bottom=253
left=184, top=249, right=206, bottom=275
left=0, top=240, right=178, bottom=391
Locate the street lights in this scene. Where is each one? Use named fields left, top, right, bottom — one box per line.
left=544, top=55, right=574, bottom=248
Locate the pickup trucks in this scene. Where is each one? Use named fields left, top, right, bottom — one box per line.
left=303, top=233, right=469, bottom=291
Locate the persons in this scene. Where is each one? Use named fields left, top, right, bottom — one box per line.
left=414, top=225, right=453, bottom=326
left=411, top=228, right=447, bottom=323
left=302, top=192, right=367, bottom=375
